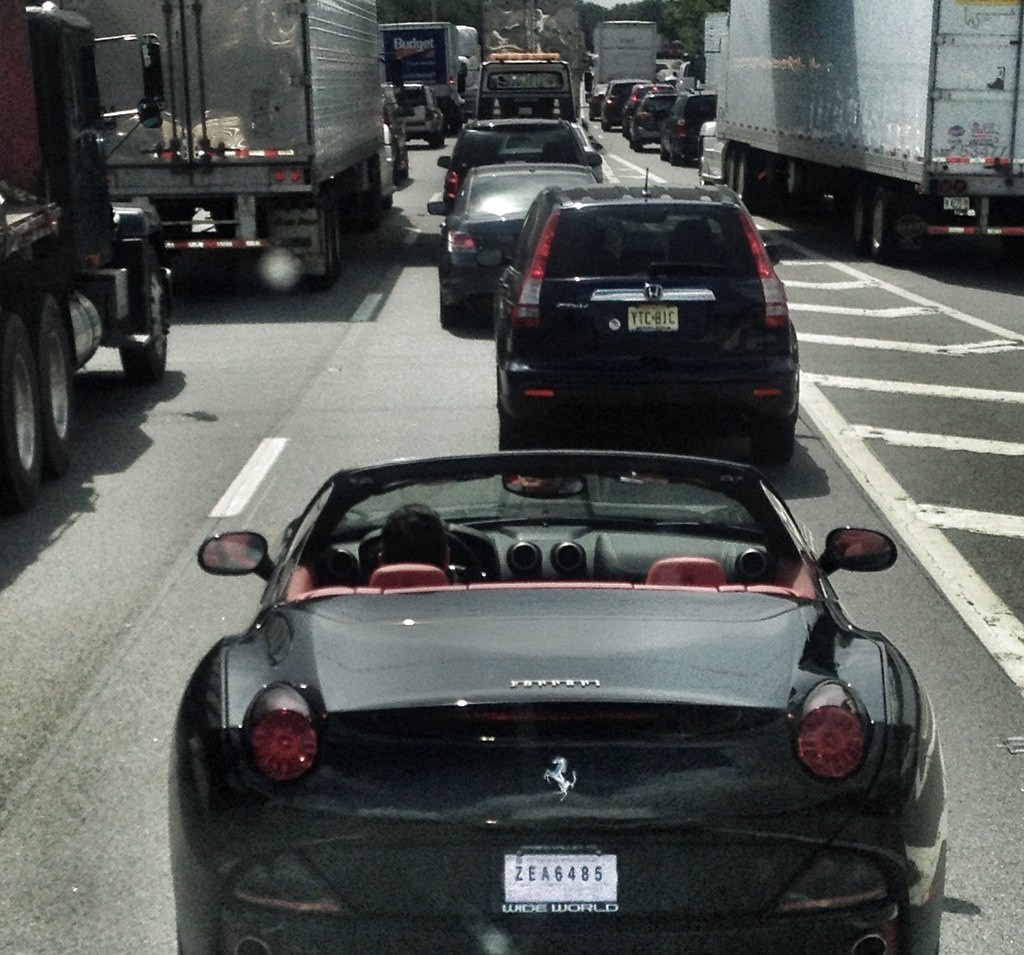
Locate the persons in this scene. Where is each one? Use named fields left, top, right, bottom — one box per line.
left=382, top=504, right=452, bottom=585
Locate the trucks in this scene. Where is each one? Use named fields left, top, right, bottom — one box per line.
left=2, top=0, right=178, bottom=524
left=710, top=0, right=1024, bottom=266
left=377, top=19, right=465, bottom=139
left=592, top=19, right=661, bottom=101
left=87, top=1, right=412, bottom=295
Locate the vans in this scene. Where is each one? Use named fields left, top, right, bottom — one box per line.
left=474, top=51, right=577, bottom=118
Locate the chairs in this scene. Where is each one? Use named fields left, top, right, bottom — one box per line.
left=366, top=561, right=453, bottom=594
left=643, top=553, right=729, bottom=594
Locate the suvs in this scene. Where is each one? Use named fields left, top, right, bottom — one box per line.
left=388, top=81, right=449, bottom=151
left=491, top=168, right=802, bottom=462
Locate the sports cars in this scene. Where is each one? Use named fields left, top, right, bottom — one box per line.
left=165, top=445, right=953, bottom=955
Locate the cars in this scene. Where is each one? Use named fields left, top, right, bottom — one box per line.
left=588, top=78, right=654, bottom=134
left=449, top=117, right=589, bottom=161
left=654, top=58, right=695, bottom=90
left=438, top=161, right=602, bottom=327
left=657, top=85, right=719, bottom=168
left=620, top=83, right=670, bottom=152
